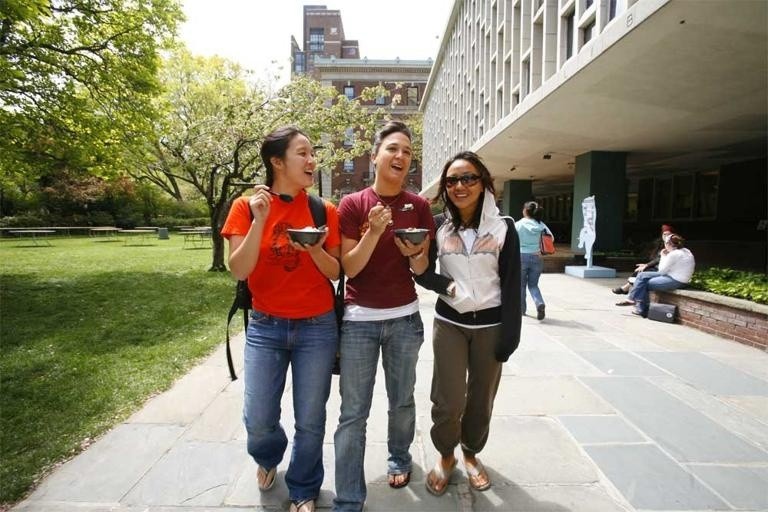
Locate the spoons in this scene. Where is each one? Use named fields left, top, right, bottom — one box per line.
left=384, top=203, right=414, bottom=212
left=267, top=190, right=293, bottom=203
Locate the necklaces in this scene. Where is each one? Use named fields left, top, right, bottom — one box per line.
left=370, top=186, right=403, bottom=208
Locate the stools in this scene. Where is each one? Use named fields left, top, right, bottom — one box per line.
left=159, top=228, right=168, bottom=239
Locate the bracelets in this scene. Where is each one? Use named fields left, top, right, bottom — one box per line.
left=410, top=248, right=424, bottom=260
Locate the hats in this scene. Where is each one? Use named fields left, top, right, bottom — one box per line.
left=661, top=225, right=673, bottom=233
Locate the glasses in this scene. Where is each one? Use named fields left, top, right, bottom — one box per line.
left=444, top=174, right=482, bottom=188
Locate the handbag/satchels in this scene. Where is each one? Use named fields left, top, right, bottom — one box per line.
left=332, top=294, right=345, bottom=375
left=647, top=301, right=677, bottom=323
left=539, top=233, right=556, bottom=255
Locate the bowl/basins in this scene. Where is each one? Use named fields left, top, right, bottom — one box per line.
left=286, top=228, right=325, bottom=248
left=391, top=227, right=429, bottom=245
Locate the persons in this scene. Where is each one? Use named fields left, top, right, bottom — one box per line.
left=218, top=125, right=341, bottom=512
left=515, top=200, right=555, bottom=320
left=411, top=150, right=523, bottom=497
left=330, top=120, right=436, bottom=512
left=614, top=234, right=696, bottom=318
left=611, top=224, right=676, bottom=295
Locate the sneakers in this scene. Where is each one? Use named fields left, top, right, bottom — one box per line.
left=615, top=299, right=636, bottom=305
left=612, top=286, right=629, bottom=294
left=632, top=308, right=645, bottom=315
left=537, top=303, right=545, bottom=320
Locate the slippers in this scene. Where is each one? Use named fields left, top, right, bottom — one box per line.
left=425, top=457, right=458, bottom=496
left=387, top=461, right=412, bottom=487
left=461, top=457, right=491, bottom=490
left=290, top=498, right=315, bottom=512
left=257, top=463, right=277, bottom=490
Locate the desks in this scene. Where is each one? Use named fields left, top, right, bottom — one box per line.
left=10, top=230, right=56, bottom=248
left=90, top=227, right=122, bottom=242
left=135, top=226, right=158, bottom=238
left=117, top=229, right=155, bottom=246
left=173, top=224, right=213, bottom=249
